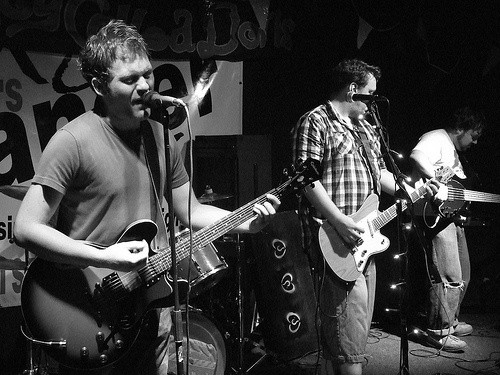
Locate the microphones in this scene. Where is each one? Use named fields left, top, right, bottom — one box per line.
left=143, top=91, right=185, bottom=105
left=346, top=91, right=388, bottom=102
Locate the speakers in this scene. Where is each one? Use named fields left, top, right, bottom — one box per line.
left=248, top=211, right=326, bottom=364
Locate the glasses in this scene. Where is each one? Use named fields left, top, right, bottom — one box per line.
left=466, top=129, right=478, bottom=141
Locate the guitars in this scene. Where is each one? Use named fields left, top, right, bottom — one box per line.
left=20, top=156, right=323, bottom=370
left=414, top=177, right=500, bottom=221
left=316, top=167, right=451, bottom=284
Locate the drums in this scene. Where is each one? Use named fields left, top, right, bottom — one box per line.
left=156, top=305, right=227, bottom=374
left=168, top=228, right=228, bottom=300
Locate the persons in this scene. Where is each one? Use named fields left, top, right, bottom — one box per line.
left=290, top=58, right=441, bottom=375
left=410, top=104, right=485, bottom=352
left=13, top=20, right=281, bottom=375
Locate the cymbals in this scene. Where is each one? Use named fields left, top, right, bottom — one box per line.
left=197, top=180, right=231, bottom=203
left=214, top=237, right=246, bottom=246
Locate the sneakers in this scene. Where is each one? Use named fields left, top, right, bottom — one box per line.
left=452, top=322, right=473, bottom=337
left=427, top=335, right=468, bottom=352
